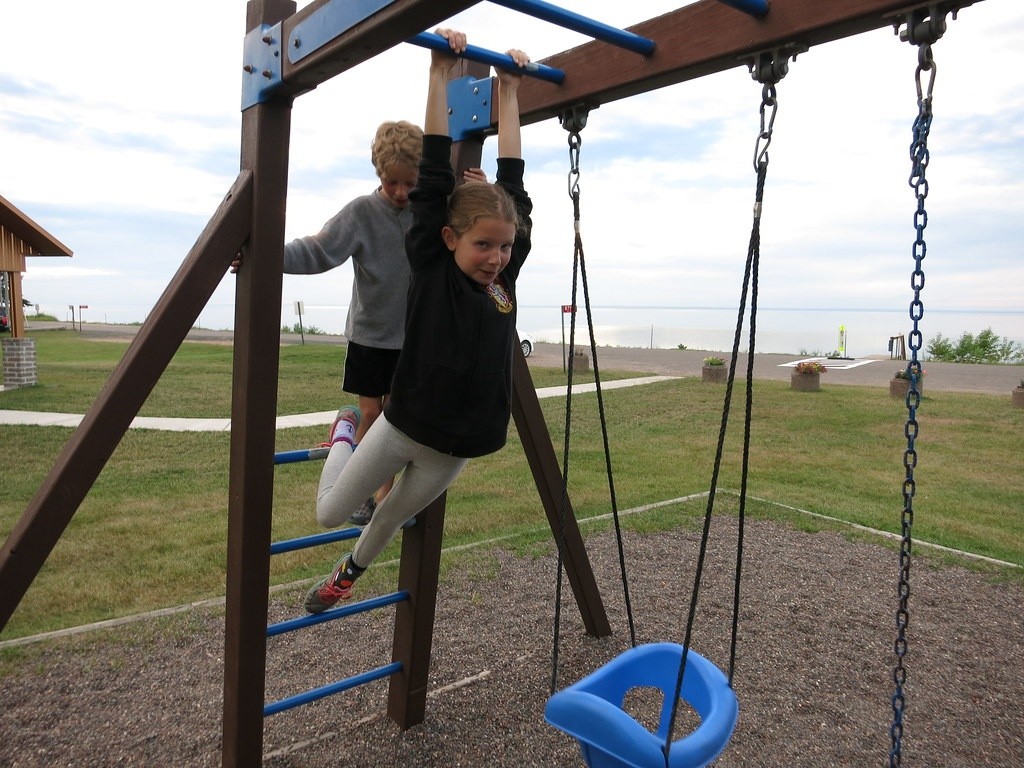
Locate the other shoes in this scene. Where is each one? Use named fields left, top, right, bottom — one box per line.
left=401, top=517, right=416, bottom=528
left=349, top=497, right=375, bottom=525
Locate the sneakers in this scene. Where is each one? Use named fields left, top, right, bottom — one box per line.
left=315, top=405, right=360, bottom=447
left=304, top=553, right=352, bottom=614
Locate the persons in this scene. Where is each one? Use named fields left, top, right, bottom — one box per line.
left=227, top=121, right=488, bottom=528
left=303, top=30, right=532, bottom=615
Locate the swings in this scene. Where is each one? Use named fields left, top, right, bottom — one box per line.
left=538, top=62, right=780, bottom=768
